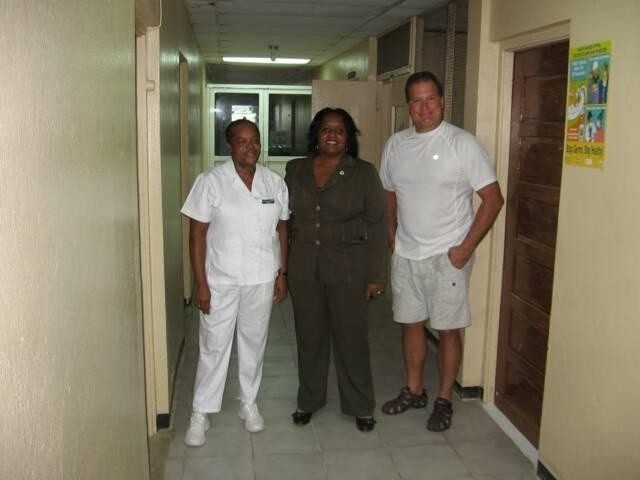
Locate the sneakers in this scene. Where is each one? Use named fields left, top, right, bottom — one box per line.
left=183, top=411, right=210, bottom=448
left=237, top=402, right=265, bottom=433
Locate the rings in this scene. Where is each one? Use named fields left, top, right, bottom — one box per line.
left=196, top=304, right=200, bottom=308
left=377, top=290, right=383, bottom=295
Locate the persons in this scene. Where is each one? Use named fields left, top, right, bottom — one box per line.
left=179, top=119, right=290, bottom=447
left=381, top=72, right=504, bottom=431
left=284, top=109, right=386, bottom=433
left=576, top=60, right=607, bottom=144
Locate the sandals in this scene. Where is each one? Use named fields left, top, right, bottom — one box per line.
left=291, top=408, right=313, bottom=426
left=355, top=414, right=375, bottom=432
left=426, top=397, right=454, bottom=432
left=381, top=385, right=428, bottom=416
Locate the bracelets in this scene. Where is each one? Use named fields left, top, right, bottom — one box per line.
left=279, top=271, right=289, bottom=277
